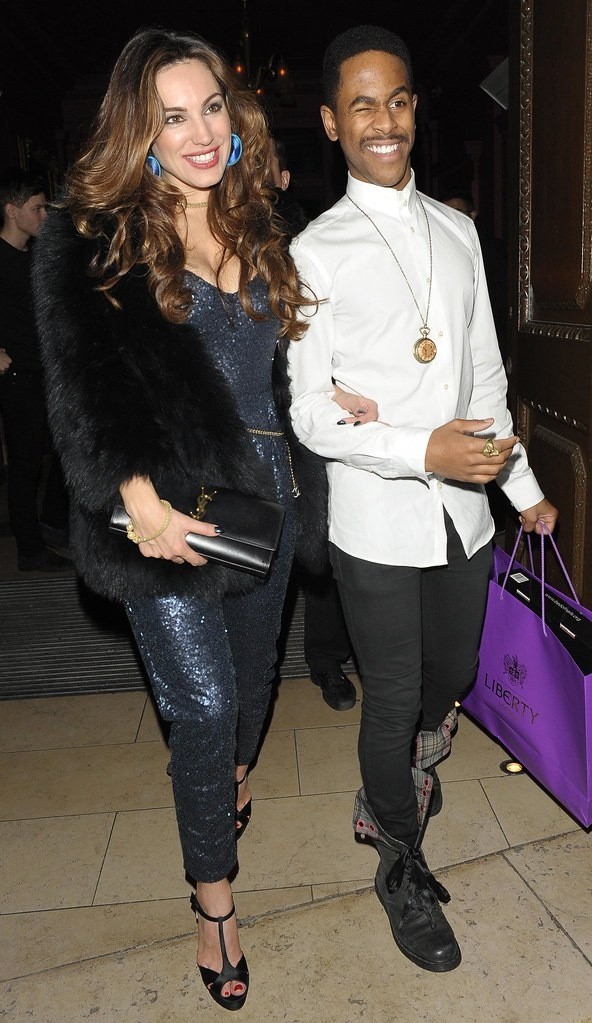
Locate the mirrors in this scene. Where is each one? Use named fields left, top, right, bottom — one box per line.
left=516, top=0, right=592, bottom=343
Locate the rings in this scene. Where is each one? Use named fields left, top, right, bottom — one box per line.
left=482, top=438, right=500, bottom=458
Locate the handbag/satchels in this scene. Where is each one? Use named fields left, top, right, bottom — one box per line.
left=108, top=465, right=286, bottom=578
left=460, top=521, right=592, bottom=828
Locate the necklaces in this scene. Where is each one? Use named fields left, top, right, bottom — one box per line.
left=177, top=201, right=208, bottom=209
left=346, top=192, right=437, bottom=364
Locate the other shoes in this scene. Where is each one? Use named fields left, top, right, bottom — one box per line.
left=18, top=549, right=73, bottom=572
left=42, top=504, right=70, bottom=530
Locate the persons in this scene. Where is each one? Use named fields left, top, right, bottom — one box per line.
left=0, top=169, right=76, bottom=572
left=440, top=190, right=507, bottom=536
left=305, top=575, right=357, bottom=711
left=33, top=31, right=317, bottom=1012
left=286, top=27, right=558, bottom=972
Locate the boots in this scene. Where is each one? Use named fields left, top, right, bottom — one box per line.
left=411, top=707, right=458, bottom=817
left=352, top=766, right=461, bottom=972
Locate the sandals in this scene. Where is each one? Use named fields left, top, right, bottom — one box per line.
left=190, top=891, right=249, bottom=1011
left=234, top=771, right=252, bottom=841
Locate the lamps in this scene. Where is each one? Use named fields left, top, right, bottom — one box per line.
left=478, top=59, right=509, bottom=110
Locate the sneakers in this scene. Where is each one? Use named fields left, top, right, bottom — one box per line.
left=311, top=666, right=356, bottom=711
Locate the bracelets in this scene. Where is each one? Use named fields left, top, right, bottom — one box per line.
left=126, top=500, right=172, bottom=544
